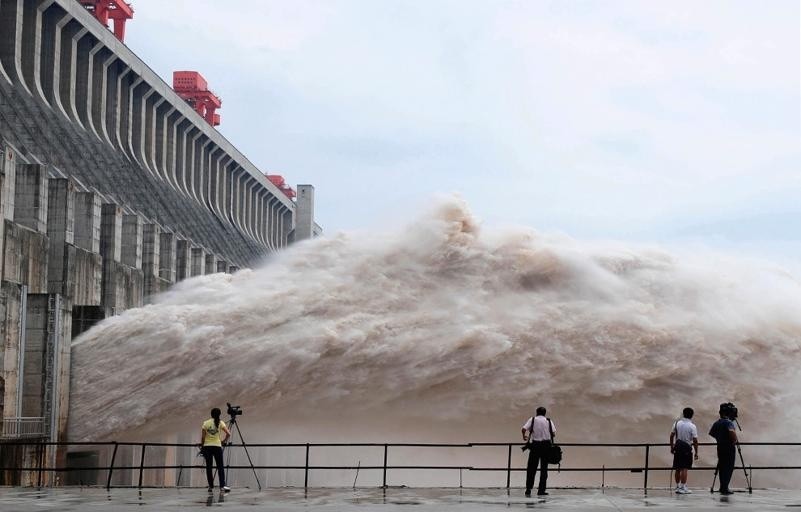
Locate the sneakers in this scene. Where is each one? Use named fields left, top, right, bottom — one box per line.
left=673, top=487, right=736, bottom=495
left=525, top=488, right=549, bottom=496
left=207, top=485, right=231, bottom=492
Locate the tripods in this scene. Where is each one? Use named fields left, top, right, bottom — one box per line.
left=710, top=434, right=757, bottom=494
left=213, top=421, right=261, bottom=492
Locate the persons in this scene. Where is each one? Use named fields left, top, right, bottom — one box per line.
left=709, top=406, right=739, bottom=494
left=521, top=406, right=557, bottom=495
left=201, top=407, right=232, bottom=492
left=670, top=407, right=700, bottom=494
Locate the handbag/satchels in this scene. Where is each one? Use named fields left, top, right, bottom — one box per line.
left=673, top=440, right=693, bottom=458
left=548, top=445, right=563, bottom=464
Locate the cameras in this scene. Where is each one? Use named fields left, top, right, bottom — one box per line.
left=519, top=443, right=533, bottom=451
